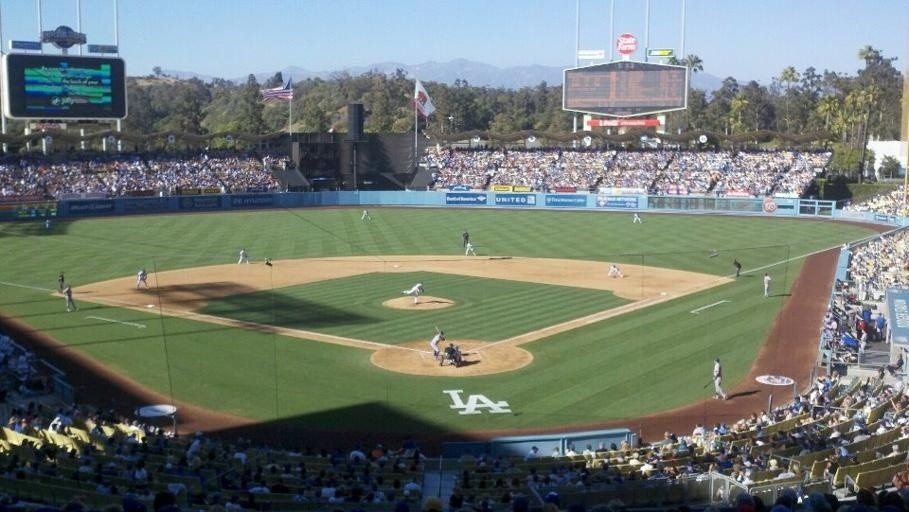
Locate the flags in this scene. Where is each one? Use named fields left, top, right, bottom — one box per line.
left=262, top=77, right=293, bottom=103
left=415, top=80, right=437, bottom=119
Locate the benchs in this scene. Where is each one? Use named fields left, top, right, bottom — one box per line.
left=1, top=328, right=908, bottom=512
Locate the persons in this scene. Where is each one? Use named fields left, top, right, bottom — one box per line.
left=4, top=147, right=909, bottom=512
